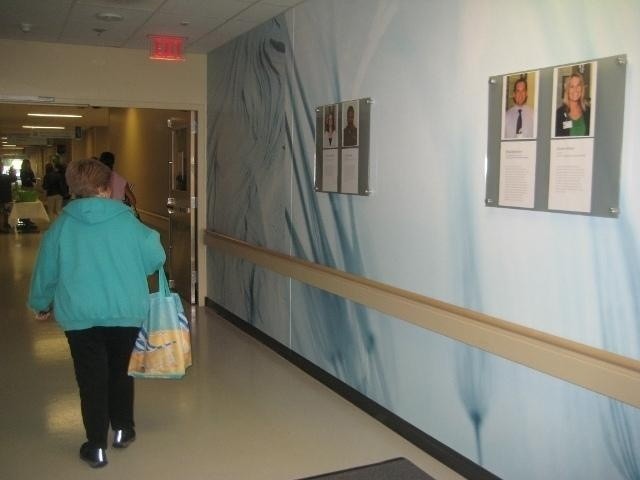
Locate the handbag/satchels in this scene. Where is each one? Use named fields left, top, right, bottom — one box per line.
left=126, top=290, right=192, bottom=381
left=56, top=179, right=68, bottom=197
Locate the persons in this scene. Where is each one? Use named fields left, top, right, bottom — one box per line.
left=20, top=159, right=34, bottom=187
left=100, top=152, right=136, bottom=205
left=503, top=79, right=535, bottom=137
left=343, top=105, right=358, bottom=146
left=42, top=163, right=64, bottom=220
left=54, top=164, right=69, bottom=199
left=9, top=166, right=16, bottom=183
left=0, top=162, right=13, bottom=233
left=27, top=159, right=192, bottom=468
left=555, top=73, right=590, bottom=136
left=322, top=113, right=338, bottom=146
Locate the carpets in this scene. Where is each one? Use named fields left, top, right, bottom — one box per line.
left=294, top=456, right=437, bottom=480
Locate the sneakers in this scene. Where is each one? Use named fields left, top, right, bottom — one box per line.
left=111, top=428, right=135, bottom=449
left=79, top=443, right=107, bottom=467
